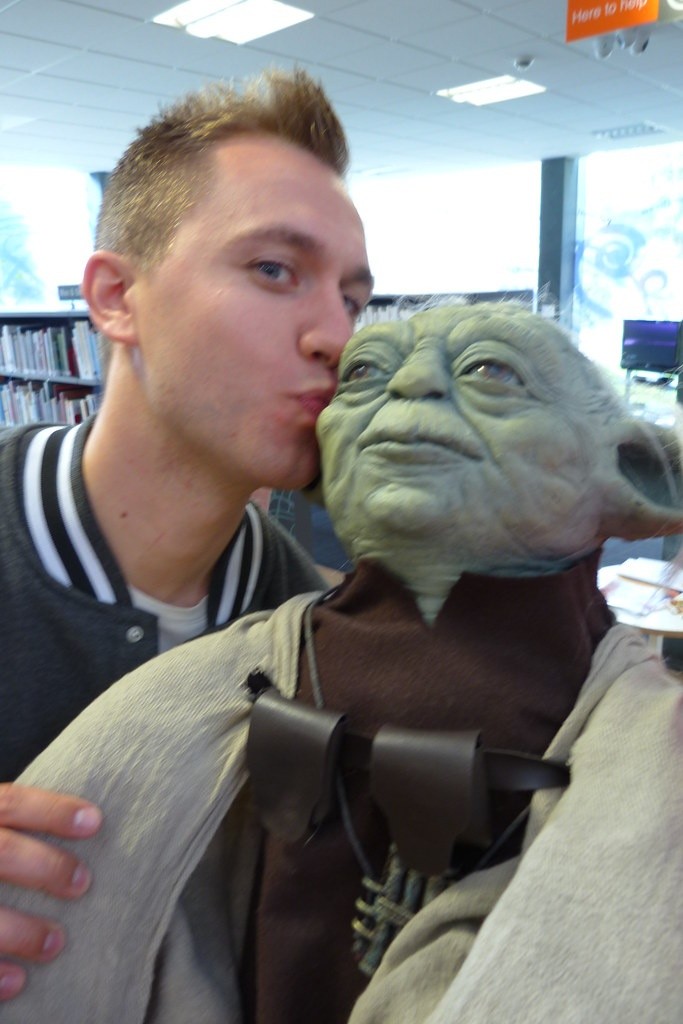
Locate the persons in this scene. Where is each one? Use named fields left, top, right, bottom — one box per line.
left=0, top=66, right=373, bottom=1005
left=0, top=301, right=683, bottom=1024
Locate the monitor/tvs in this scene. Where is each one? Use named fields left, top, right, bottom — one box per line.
left=621, top=321, right=683, bottom=373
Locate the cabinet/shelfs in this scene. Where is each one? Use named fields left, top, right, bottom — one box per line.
left=0, top=292, right=537, bottom=427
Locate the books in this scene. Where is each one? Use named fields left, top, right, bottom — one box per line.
left=0, top=320, right=101, bottom=427
